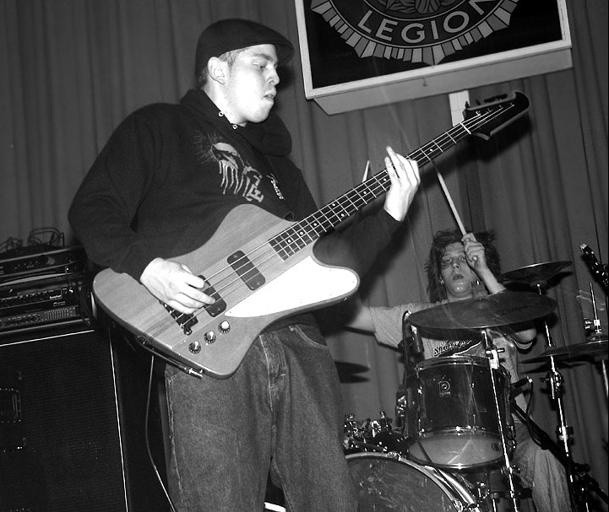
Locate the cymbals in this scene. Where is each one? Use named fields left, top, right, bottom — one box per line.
left=405, top=290, right=558, bottom=331
left=518, top=342, right=604, bottom=365
left=333, top=358, right=367, bottom=378
left=497, top=258, right=574, bottom=288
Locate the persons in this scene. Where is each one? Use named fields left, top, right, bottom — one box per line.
left=67, top=18, right=421, bottom=512
left=343, top=229, right=572, bottom=512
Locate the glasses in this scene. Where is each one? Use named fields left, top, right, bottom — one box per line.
left=439, top=255, right=467, bottom=265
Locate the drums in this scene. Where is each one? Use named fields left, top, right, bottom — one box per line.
left=339, top=418, right=494, bottom=512
left=400, top=355, right=518, bottom=473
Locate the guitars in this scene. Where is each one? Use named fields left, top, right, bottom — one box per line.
left=91, top=88, right=532, bottom=378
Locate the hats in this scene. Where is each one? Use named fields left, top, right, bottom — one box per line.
left=195, top=19, right=295, bottom=74
